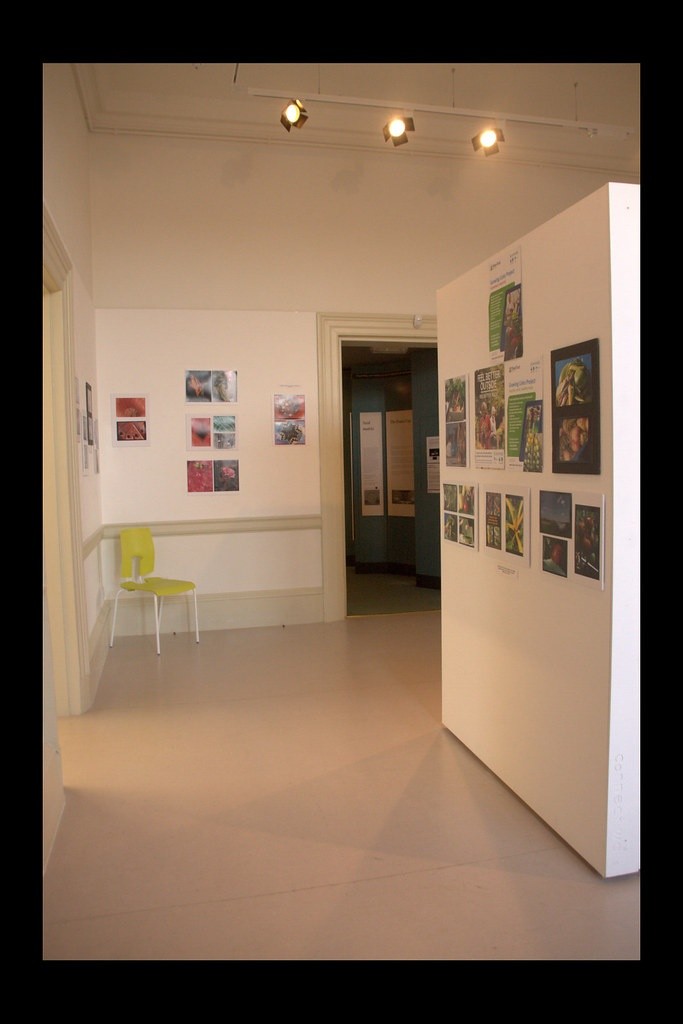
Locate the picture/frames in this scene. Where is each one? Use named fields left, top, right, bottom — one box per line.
left=441, top=481, right=532, bottom=568
left=534, top=486, right=605, bottom=591
left=551, top=338, right=601, bottom=475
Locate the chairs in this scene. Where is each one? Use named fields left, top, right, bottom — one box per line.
left=109, top=528, right=199, bottom=655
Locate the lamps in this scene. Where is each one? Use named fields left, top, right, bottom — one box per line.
left=383, top=114, right=414, bottom=147
left=280, top=98, right=309, bottom=132
left=471, top=127, right=506, bottom=157
